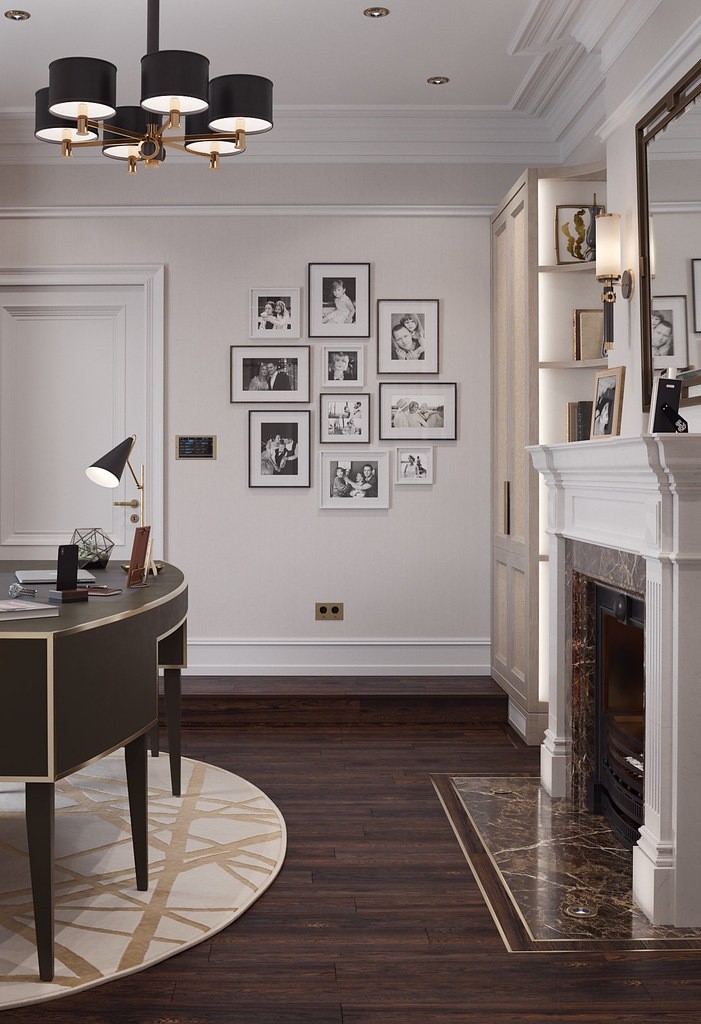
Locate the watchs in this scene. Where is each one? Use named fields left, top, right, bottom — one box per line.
left=8, top=583, right=38, bottom=598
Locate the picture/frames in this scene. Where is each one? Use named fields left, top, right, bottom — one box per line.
left=691, top=259, right=701, bottom=333
left=647, top=377, right=689, bottom=433
left=377, top=299, right=439, bottom=374
left=84, top=434, right=164, bottom=576
left=249, top=287, right=301, bottom=338
left=248, top=410, right=311, bottom=488
left=319, top=392, right=371, bottom=444
left=229, top=344, right=311, bottom=403
left=650, top=295, right=689, bottom=371
left=589, top=366, right=626, bottom=440
left=378, top=381, right=457, bottom=441
left=395, top=446, right=433, bottom=484
left=319, top=451, right=389, bottom=509
left=307, top=262, right=371, bottom=338
left=321, top=345, right=365, bottom=387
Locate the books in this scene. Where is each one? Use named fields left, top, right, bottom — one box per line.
left=573, top=308, right=604, bottom=359
left=568, top=401, right=592, bottom=441
left=0, top=599, right=59, bottom=621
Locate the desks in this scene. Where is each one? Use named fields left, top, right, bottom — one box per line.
left=0, top=560, right=188, bottom=982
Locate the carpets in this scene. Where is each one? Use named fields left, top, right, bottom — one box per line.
left=0, top=748, right=288, bottom=1013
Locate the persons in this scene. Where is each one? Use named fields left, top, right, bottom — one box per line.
left=652, top=311, right=672, bottom=355
left=394, top=397, right=435, bottom=429
left=341, top=402, right=361, bottom=435
left=259, top=300, right=289, bottom=329
left=249, top=362, right=292, bottom=390
left=332, top=464, right=378, bottom=497
left=594, top=399, right=609, bottom=434
left=329, top=352, right=352, bottom=379
left=323, top=279, right=354, bottom=324
left=261, top=431, right=299, bottom=474
left=392, top=313, right=425, bottom=360
left=404, top=455, right=422, bottom=477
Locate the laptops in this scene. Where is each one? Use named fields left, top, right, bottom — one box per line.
left=14, top=569, right=96, bottom=583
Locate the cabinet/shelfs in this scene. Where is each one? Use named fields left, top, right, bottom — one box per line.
left=491, top=166, right=554, bottom=745
left=538, top=260, right=608, bottom=369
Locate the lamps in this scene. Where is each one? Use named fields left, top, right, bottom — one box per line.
left=596, top=212, right=635, bottom=298
left=34, top=0, right=274, bottom=173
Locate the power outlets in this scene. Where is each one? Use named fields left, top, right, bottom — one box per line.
left=315, top=603, right=344, bottom=620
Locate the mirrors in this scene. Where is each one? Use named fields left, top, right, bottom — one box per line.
left=634, top=60, right=701, bottom=412
